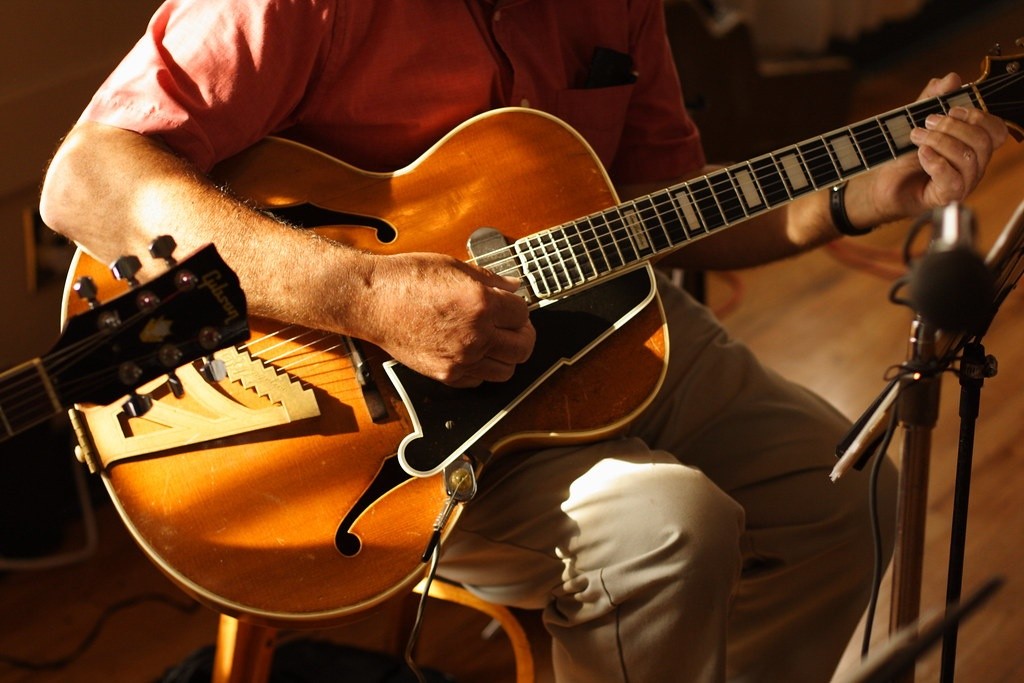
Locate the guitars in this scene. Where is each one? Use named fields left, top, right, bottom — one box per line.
left=0, top=234, right=250, bottom=434
left=60, top=35, right=1024, bottom=628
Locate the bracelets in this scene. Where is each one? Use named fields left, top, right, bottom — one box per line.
left=829, top=180, right=879, bottom=235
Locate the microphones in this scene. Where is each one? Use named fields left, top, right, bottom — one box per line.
left=908, top=241, right=996, bottom=336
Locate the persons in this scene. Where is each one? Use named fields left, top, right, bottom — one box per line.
left=40, top=0, right=1009, bottom=683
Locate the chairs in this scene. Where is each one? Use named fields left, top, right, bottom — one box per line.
left=212, top=578, right=556, bottom=683
